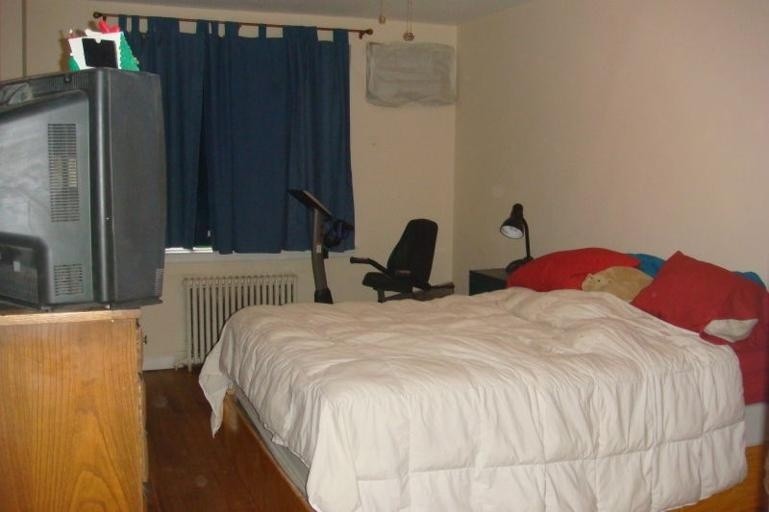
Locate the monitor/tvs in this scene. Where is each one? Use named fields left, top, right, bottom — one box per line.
left=0, top=66, right=168, bottom=312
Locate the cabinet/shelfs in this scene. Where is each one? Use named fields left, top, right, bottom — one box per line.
left=469, top=268, right=510, bottom=296
left=0, top=308, right=149, bottom=512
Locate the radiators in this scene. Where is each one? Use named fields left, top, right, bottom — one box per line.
left=174, top=272, right=299, bottom=374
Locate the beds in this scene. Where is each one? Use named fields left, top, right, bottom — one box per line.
left=222, top=262, right=768, bottom=511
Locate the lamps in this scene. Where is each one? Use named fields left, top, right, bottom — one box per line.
left=500, top=203, right=534, bottom=272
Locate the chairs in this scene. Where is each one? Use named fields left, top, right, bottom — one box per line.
left=350, top=218, right=438, bottom=302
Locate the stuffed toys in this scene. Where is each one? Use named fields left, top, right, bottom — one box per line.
left=581, top=264, right=655, bottom=306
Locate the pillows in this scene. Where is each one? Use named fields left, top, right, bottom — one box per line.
left=505, top=247, right=761, bottom=346
